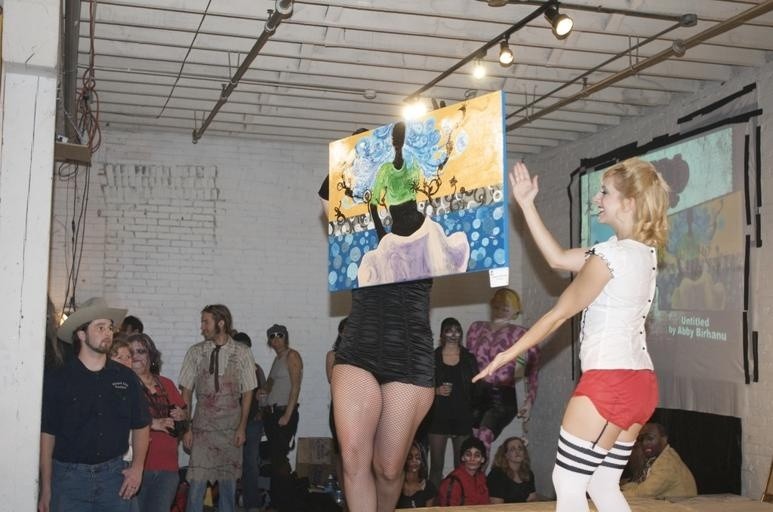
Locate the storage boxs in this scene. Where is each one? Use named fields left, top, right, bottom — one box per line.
left=296, top=436, right=341, bottom=491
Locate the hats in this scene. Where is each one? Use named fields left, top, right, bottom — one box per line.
left=55, top=297, right=129, bottom=346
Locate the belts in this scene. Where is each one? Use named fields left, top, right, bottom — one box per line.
left=262, top=406, right=298, bottom=414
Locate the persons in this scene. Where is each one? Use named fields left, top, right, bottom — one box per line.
left=487, top=436, right=537, bottom=504
left=359, top=122, right=470, bottom=288
left=395, top=440, right=437, bottom=508
left=439, top=436, right=491, bottom=507
left=466, top=286, right=540, bottom=474
left=326, top=316, right=348, bottom=492
left=426, top=318, right=479, bottom=488
left=471, top=156, right=671, bottom=512
left=318, top=98, right=446, bottom=512
left=620, top=420, right=698, bottom=497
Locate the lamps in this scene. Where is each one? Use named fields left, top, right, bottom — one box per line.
left=392, top=2, right=574, bottom=127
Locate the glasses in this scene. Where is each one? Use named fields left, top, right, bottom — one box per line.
left=268, top=333, right=285, bottom=340
